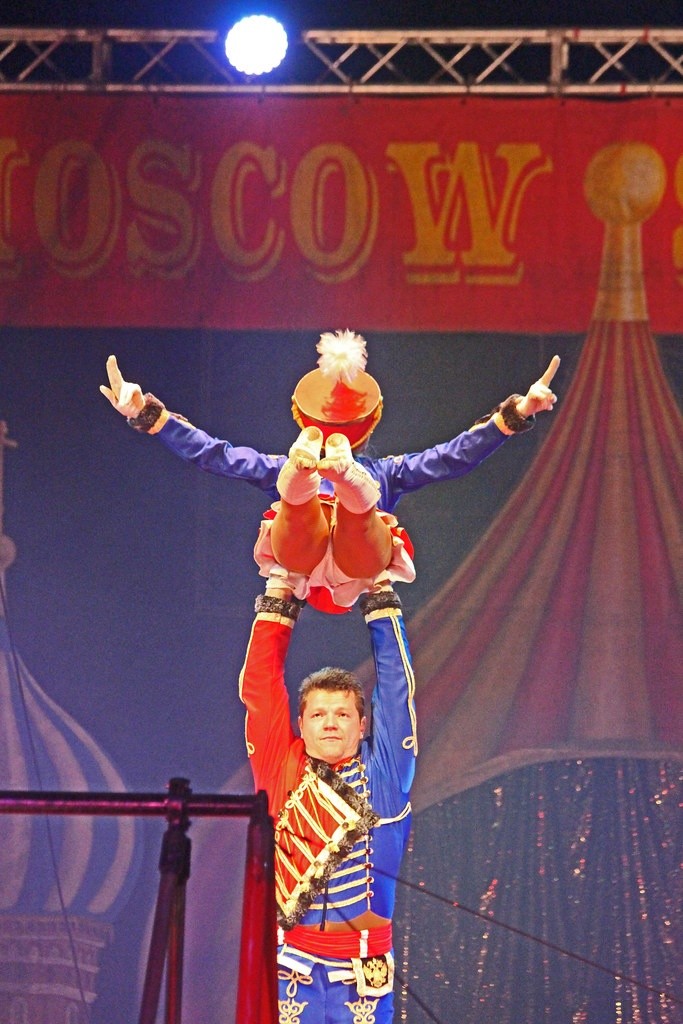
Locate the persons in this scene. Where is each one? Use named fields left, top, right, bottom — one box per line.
left=100, top=329, right=562, bottom=615
left=237, top=570, right=419, bottom=1024
